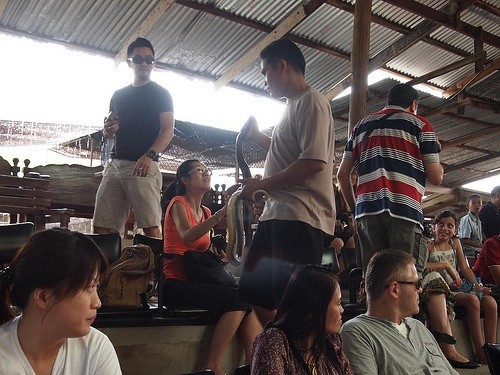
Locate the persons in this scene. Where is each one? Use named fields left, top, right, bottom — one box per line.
left=235, top=38, right=335, bottom=322
left=328, top=173, right=500, bottom=368
left=92, top=36, right=175, bottom=238
left=160, top=160, right=263, bottom=375
left=337, top=83, right=443, bottom=298
left=250, top=264, right=355, bottom=375
left=339, top=249, right=461, bottom=375
left=0, top=226, right=123, bottom=375
left=421, top=209, right=498, bottom=356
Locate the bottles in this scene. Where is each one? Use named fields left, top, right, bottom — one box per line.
left=473, top=277, right=483, bottom=300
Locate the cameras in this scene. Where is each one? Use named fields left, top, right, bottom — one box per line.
left=106, top=111, right=120, bottom=124
left=212, top=235, right=227, bottom=254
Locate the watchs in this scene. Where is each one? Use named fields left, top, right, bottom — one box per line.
left=146, top=150, right=160, bottom=161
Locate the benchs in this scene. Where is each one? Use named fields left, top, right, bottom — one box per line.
left=0, top=174, right=76, bottom=232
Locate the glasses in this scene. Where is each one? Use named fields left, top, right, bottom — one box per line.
left=127, top=55, right=154, bottom=64
left=385, top=279, right=422, bottom=291
left=181, top=167, right=213, bottom=178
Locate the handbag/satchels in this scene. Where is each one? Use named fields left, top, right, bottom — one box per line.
left=184, top=250, right=236, bottom=286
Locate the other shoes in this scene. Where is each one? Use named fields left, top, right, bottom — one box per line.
left=447, top=357, right=481, bottom=369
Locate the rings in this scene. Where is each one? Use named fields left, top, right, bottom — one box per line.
left=140, top=168, right=143, bottom=170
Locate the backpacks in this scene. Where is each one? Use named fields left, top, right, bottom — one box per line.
left=97, top=245, right=155, bottom=313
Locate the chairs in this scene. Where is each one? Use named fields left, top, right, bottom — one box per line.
left=0, top=221, right=35, bottom=325
left=86, top=231, right=156, bottom=319
left=134, top=234, right=214, bottom=318
